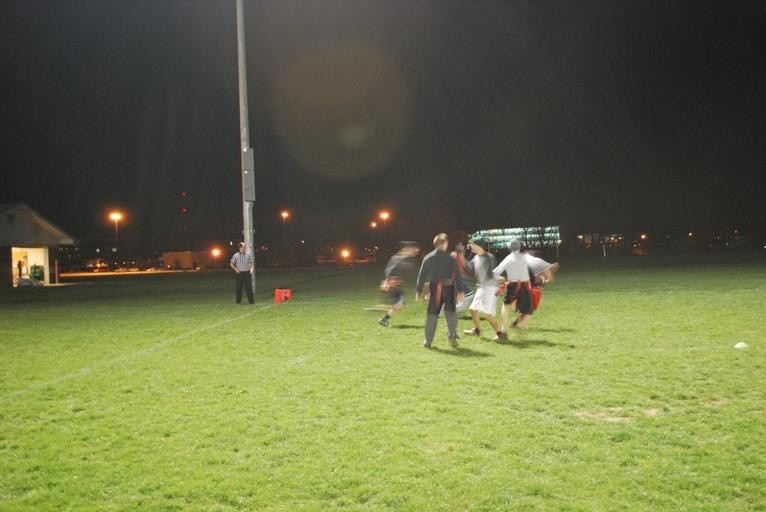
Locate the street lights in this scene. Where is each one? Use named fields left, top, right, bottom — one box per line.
left=109, top=211, right=124, bottom=261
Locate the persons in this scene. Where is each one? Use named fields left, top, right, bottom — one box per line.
left=230, top=242, right=254, bottom=304
left=364, top=232, right=560, bottom=349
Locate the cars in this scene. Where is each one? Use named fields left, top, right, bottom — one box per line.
left=137, top=257, right=165, bottom=272
left=259, top=235, right=318, bottom=269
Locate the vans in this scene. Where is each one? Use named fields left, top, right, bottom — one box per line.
left=71, top=241, right=109, bottom=271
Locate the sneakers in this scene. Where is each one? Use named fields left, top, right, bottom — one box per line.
left=464, top=327, right=479, bottom=336
left=422, top=341, right=432, bottom=348
left=493, top=333, right=508, bottom=340
left=378, top=317, right=389, bottom=327
left=448, top=339, right=457, bottom=345
